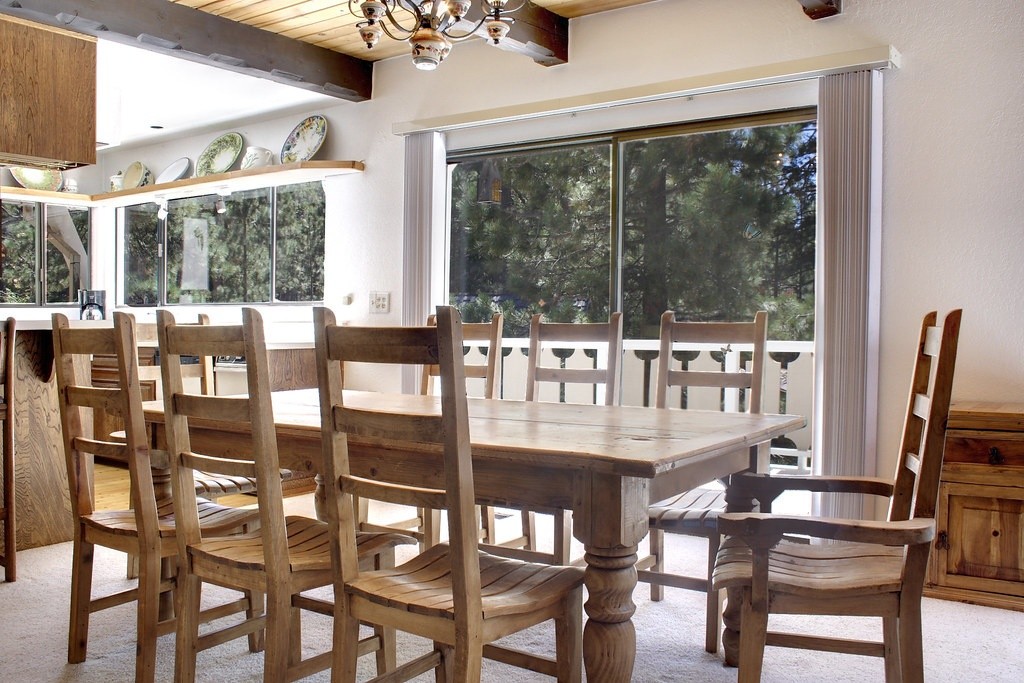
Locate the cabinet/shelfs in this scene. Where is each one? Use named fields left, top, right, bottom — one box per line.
left=0, top=13, right=98, bottom=173
left=88, top=350, right=157, bottom=473
left=920, top=398, right=1024, bottom=614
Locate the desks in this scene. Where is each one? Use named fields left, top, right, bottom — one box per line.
left=141, top=388, right=807, bottom=683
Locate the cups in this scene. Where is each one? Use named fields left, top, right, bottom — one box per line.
left=110, top=171, right=123, bottom=192
left=240, top=146, right=273, bottom=170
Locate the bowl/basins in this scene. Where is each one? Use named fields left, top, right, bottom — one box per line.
left=122, top=160, right=153, bottom=190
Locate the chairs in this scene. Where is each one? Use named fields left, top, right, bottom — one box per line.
left=0, top=306, right=963, bottom=683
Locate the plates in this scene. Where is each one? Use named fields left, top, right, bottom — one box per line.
left=195, top=132, right=243, bottom=177
left=155, top=157, right=190, bottom=185
left=8, top=168, right=63, bottom=192
left=280, top=114, right=328, bottom=163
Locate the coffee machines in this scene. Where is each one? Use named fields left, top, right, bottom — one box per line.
left=77, top=290, right=106, bottom=321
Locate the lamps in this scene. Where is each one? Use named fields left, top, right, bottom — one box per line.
left=347, top=0, right=526, bottom=71
